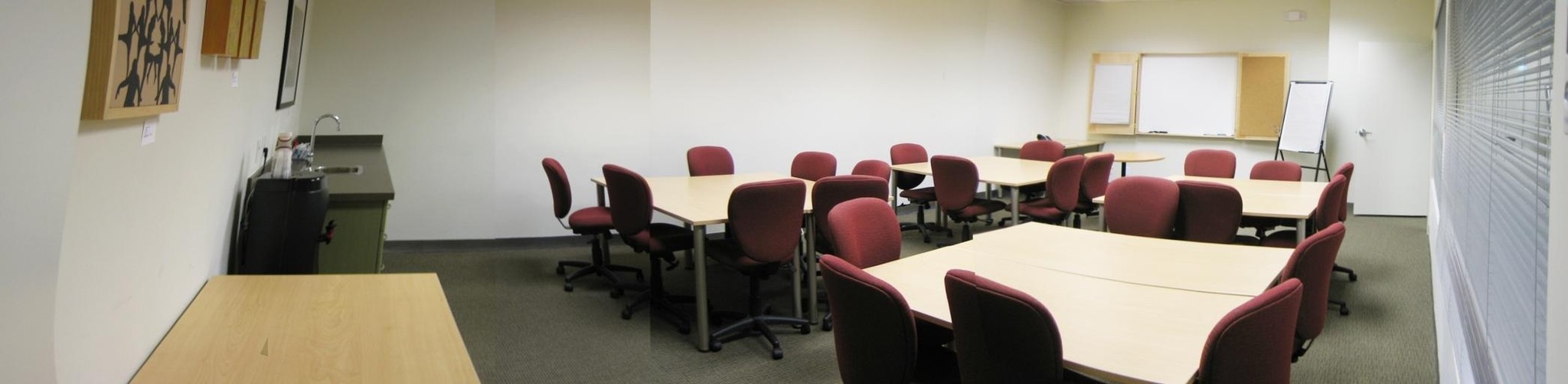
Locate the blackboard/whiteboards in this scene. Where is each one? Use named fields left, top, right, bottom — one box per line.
left=1088, top=52, right=1288, bottom=141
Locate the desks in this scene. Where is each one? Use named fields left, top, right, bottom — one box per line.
left=127, top=271, right=481, bottom=384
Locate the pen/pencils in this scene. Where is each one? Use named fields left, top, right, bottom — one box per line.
left=1204, top=133, right=1227, bottom=136
left=1149, top=131, right=1167, bottom=133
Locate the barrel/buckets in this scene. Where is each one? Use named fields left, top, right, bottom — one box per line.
left=237, top=171, right=330, bottom=274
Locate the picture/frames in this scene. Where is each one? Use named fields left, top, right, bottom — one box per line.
left=275, top=0, right=309, bottom=110
left=79, top=0, right=193, bottom=122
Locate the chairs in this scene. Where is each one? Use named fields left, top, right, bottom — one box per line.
left=543, top=136, right=1356, bottom=384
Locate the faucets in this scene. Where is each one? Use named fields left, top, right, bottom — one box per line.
left=324, top=219, right=337, bottom=246
left=308, top=112, right=342, bottom=162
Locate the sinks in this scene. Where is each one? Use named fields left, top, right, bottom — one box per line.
left=305, top=164, right=364, bottom=176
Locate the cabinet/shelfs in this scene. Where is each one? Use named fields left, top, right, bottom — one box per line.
left=318, top=202, right=390, bottom=277
